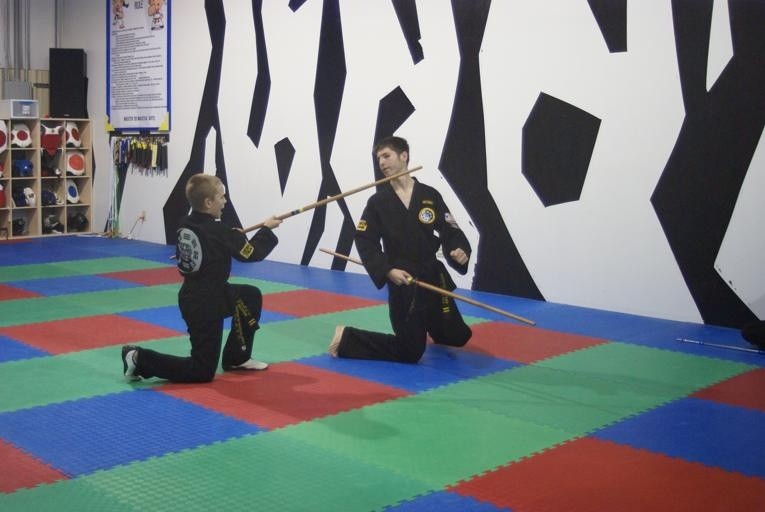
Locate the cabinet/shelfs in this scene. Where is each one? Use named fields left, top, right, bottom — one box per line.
left=0, top=118, right=94, bottom=241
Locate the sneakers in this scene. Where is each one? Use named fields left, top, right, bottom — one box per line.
left=222, top=358, right=267, bottom=370
left=122, top=346, right=141, bottom=380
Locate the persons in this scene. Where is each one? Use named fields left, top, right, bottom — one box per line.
left=122, top=174, right=283, bottom=384
left=328, top=137, right=472, bottom=364
left=112, top=0, right=125, bottom=29
left=148, top=0, right=164, bottom=30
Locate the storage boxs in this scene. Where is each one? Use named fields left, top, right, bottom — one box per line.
left=0, top=99, right=40, bottom=117
